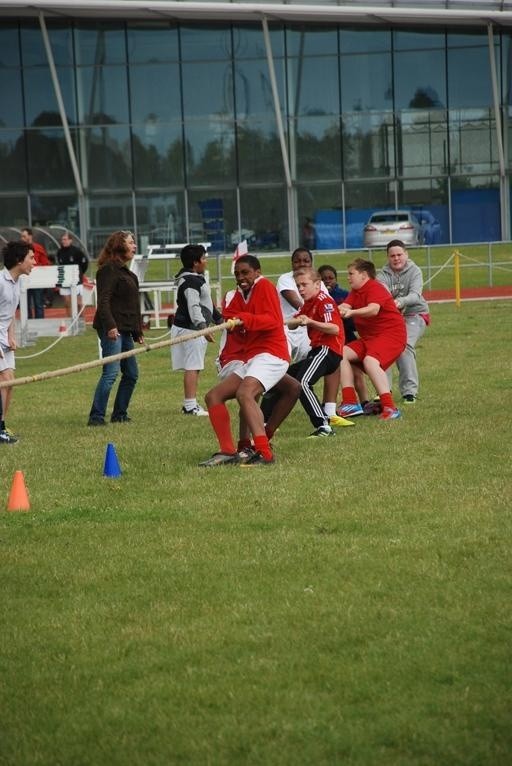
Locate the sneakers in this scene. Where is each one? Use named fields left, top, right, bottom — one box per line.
left=0, top=430, right=19, bottom=445
left=336, top=402, right=365, bottom=418
left=180, top=405, right=209, bottom=416
left=198, top=450, right=242, bottom=466
left=226, top=447, right=257, bottom=464
left=378, top=406, right=401, bottom=421
left=244, top=451, right=276, bottom=465
left=402, top=393, right=417, bottom=405
left=329, top=416, right=356, bottom=427
left=304, top=425, right=336, bottom=439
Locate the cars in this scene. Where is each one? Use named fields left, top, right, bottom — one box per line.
left=363, top=210, right=423, bottom=248
left=414, top=211, right=441, bottom=244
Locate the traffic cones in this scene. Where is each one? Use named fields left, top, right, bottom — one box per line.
left=103, top=444, right=122, bottom=478
left=7, top=471, right=31, bottom=511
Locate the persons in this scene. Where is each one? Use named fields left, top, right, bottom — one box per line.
left=0, top=241, right=36, bottom=444
left=57, top=233, right=89, bottom=318
left=215, top=289, right=301, bottom=450
left=276, top=248, right=355, bottom=428
left=374, top=239, right=430, bottom=404
left=170, top=244, right=223, bottom=417
left=20, top=227, right=50, bottom=319
left=336, top=259, right=407, bottom=420
left=87, top=230, right=144, bottom=426
left=198, top=255, right=290, bottom=468
left=288, top=269, right=345, bottom=440
left=317, top=265, right=369, bottom=407
